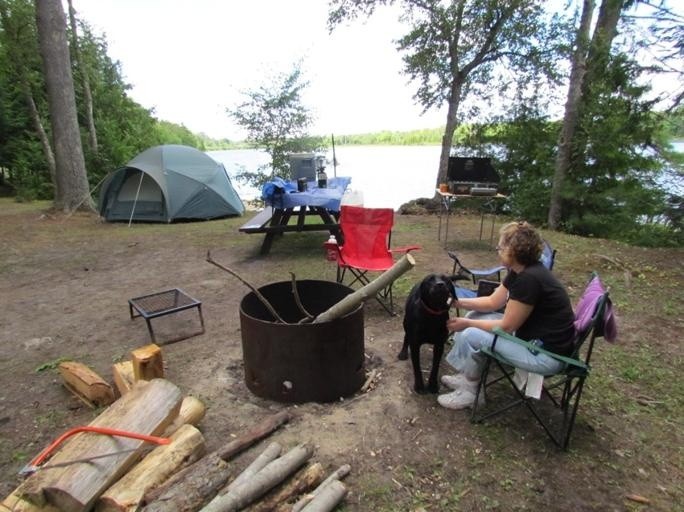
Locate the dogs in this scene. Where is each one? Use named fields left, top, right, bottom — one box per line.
left=398, top=274, right=458, bottom=395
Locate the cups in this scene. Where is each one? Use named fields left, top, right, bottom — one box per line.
left=440, top=183, right=447, bottom=192
left=297, top=178, right=307, bottom=191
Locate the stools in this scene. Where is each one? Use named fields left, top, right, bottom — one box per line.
left=127, top=287, right=206, bottom=347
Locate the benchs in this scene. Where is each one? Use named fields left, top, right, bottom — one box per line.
left=239, top=204, right=275, bottom=233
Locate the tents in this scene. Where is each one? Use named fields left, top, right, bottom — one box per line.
left=98, top=142, right=246, bottom=224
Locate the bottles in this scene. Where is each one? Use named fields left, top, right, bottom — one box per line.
left=328, top=235, right=337, bottom=244
left=317, top=168, right=326, bottom=188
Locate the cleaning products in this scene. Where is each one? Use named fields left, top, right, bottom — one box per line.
left=317, top=167, right=327, bottom=188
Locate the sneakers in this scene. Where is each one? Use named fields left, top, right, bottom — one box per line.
left=441, top=374, right=480, bottom=390
left=437, top=386, right=486, bottom=409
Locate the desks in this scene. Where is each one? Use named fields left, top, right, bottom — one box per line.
left=264, top=176, right=346, bottom=255
left=435, top=189, right=505, bottom=244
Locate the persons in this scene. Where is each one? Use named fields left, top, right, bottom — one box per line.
left=433, top=222, right=576, bottom=411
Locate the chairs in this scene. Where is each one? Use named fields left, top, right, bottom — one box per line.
left=325, top=204, right=422, bottom=317
left=443, top=240, right=613, bottom=453
